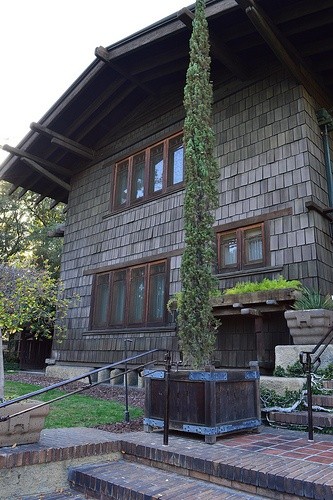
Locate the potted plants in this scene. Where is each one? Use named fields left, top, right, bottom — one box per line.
left=284, top=286, right=332, bottom=346
left=140, top=28, right=262, bottom=445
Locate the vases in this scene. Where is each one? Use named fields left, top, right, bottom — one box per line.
left=0, top=395, right=50, bottom=445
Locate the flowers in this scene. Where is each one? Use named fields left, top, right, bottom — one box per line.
left=0, top=266, right=81, bottom=343
